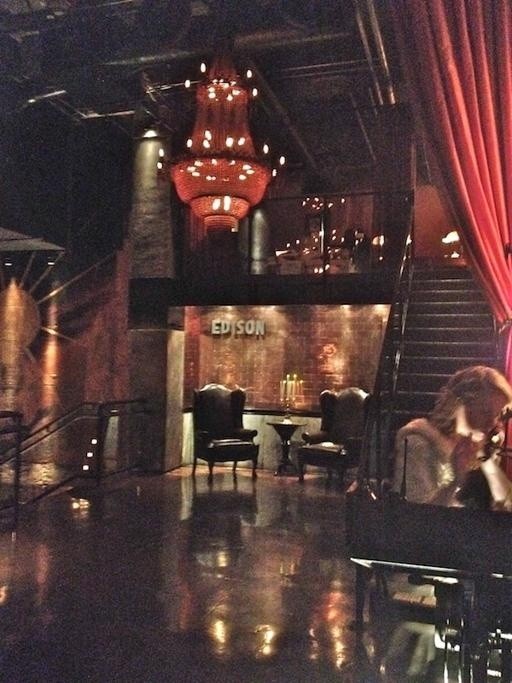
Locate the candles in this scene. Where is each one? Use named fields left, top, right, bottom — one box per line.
left=279, top=373, right=304, bottom=401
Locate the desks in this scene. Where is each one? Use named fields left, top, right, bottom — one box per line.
left=268, top=420, right=307, bottom=476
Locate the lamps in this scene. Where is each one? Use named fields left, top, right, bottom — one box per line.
left=156, top=1, right=288, bottom=234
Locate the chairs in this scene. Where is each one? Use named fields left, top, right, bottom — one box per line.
left=190, top=383, right=260, bottom=485
left=296, top=387, right=370, bottom=486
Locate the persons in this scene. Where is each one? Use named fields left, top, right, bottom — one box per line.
left=389, top=359, right=511, bottom=656
